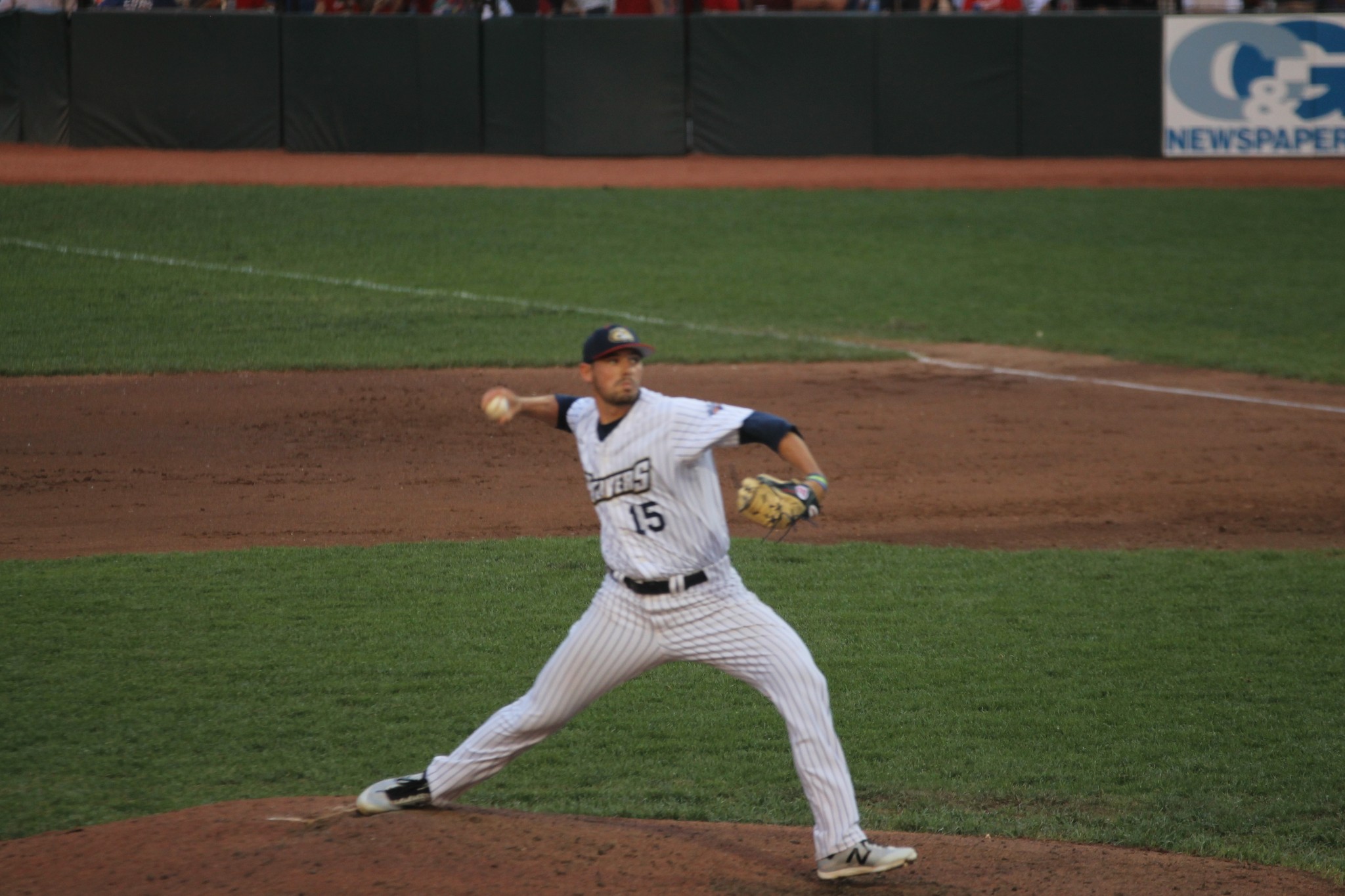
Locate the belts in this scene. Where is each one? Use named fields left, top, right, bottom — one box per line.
left=624, top=571, right=707, bottom=594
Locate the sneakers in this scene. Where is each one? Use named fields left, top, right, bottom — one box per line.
left=355, top=772, right=432, bottom=817
left=816, top=838, right=917, bottom=880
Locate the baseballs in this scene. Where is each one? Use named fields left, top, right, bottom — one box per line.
left=485, top=396, right=509, bottom=417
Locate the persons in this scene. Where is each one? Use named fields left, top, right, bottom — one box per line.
left=356, top=324, right=918, bottom=880
left=0, top=0, right=1345, bottom=15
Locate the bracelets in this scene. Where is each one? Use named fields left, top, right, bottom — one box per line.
left=805, top=473, right=828, bottom=491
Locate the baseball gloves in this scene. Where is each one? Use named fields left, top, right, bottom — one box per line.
left=737, top=473, right=822, bottom=528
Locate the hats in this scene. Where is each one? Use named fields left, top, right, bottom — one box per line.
left=583, top=324, right=655, bottom=365
